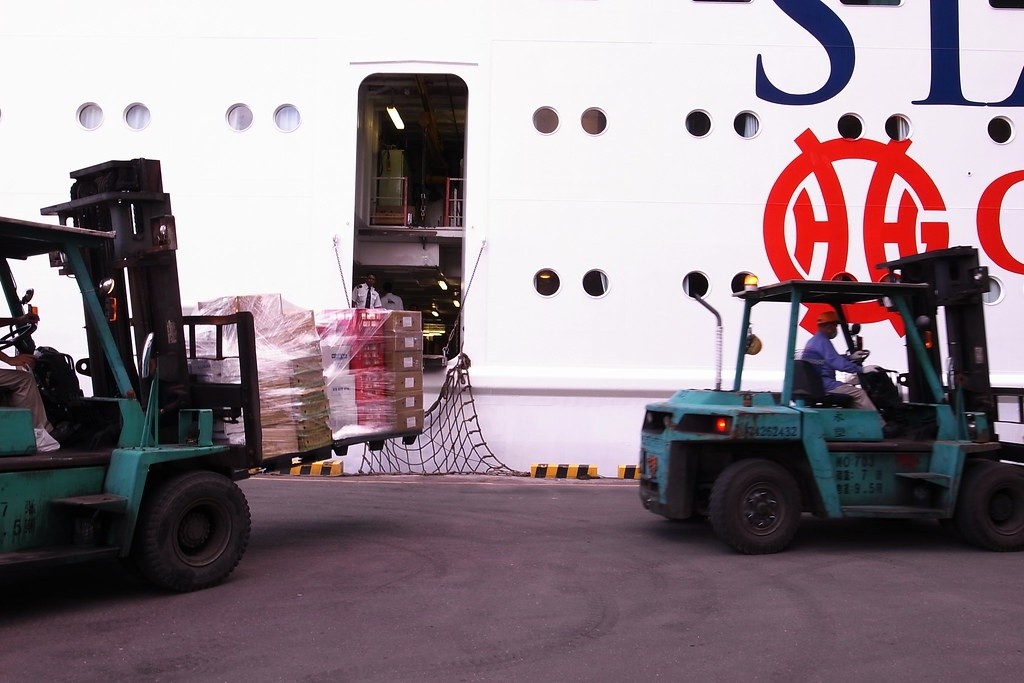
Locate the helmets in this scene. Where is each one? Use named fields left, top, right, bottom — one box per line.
left=818, top=311, right=846, bottom=325
left=743, top=333, right=762, bottom=355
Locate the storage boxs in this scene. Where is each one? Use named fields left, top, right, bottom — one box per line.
left=180, top=295, right=424, bottom=447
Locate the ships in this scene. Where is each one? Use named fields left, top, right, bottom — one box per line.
left=1, top=0, right=1024, bottom=472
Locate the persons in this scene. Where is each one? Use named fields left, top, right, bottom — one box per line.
left=381, top=282, right=403, bottom=309
left=353, top=275, right=382, bottom=308
left=801, top=312, right=906, bottom=433
left=0, top=314, right=71, bottom=439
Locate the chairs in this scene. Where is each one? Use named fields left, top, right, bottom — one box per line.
left=790, top=360, right=856, bottom=409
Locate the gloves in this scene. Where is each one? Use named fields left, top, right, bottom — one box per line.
left=849, top=350, right=868, bottom=362
left=862, top=365, right=880, bottom=373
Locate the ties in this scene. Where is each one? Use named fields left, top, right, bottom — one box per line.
left=365, top=288, right=371, bottom=308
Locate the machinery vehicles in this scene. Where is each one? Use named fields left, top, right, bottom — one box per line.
left=0, top=159, right=346, bottom=595
left=637, top=241, right=1024, bottom=553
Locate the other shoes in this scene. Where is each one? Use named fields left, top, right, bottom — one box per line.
left=49, top=420, right=73, bottom=443
left=883, top=425, right=900, bottom=435
